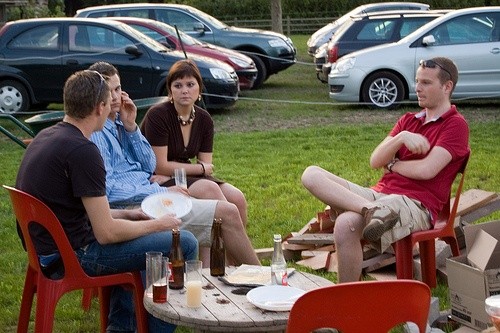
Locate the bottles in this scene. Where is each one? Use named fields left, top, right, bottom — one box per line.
left=210, top=218, right=226, bottom=276
left=270, top=233, right=288, bottom=286
left=169, top=228, right=185, bottom=289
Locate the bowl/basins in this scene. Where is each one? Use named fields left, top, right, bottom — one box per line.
left=484, top=294, right=500, bottom=331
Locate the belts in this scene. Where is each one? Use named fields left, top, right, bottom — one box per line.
left=40, top=254, right=62, bottom=271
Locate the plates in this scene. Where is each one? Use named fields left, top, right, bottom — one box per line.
left=141, top=191, right=193, bottom=220
left=247, top=285, right=308, bottom=311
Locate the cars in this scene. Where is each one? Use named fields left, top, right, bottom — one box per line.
left=327, top=6, right=500, bottom=111
left=72, top=3, right=299, bottom=89
left=305, top=1, right=492, bottom=83
left=48, top=17, right=257, bottom=91
left=1, top=17, right=240, bottom=120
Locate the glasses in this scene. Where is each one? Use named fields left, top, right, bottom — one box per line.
left=419, top=58, right=452, bottom=81
left=84, top=69, right=105, bottom=106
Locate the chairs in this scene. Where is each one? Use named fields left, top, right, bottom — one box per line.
left=0, top=184, right=146, bottom=333
left=393, top=147, right=471, bottom=288
left=286, top=278, right=432, bottom=333
left=74, top=31, right=88, bottom=48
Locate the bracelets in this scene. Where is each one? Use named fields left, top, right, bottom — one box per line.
left=200, top=163, right=205, bottom=176
left=387, top=158, right=399, bottom=172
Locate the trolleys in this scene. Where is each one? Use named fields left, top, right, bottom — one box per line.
left=1, top=96, right=175, bottom=151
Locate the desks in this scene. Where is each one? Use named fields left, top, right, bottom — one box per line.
left=141, top=264, right=337, bottom=333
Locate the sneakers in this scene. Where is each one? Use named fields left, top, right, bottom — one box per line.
left=361, top=204, right=399, bottom=241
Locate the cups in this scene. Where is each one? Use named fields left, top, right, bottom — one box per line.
left=175, top=168, right=187, bottom=190
left=145, top=251, right=162, bottom=298
left=185, top=260, right=202, bottom=308
left=152, top=257, right=169, bottom=303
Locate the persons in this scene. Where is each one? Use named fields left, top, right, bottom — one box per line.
left=138, top=59, right=248, bottom=233
left=14, top=70, right=198, bottom=333
left=86, top=62, right=261, bottom=269
left=301, top=58, right=469, bottom=284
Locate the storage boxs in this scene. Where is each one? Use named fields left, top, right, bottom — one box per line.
left=428, top=296, right=442, bottom=326
left=444, top=219, right=500, bottom=331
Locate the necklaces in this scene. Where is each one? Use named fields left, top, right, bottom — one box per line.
left=178, top=105, right=195, bottom=125
